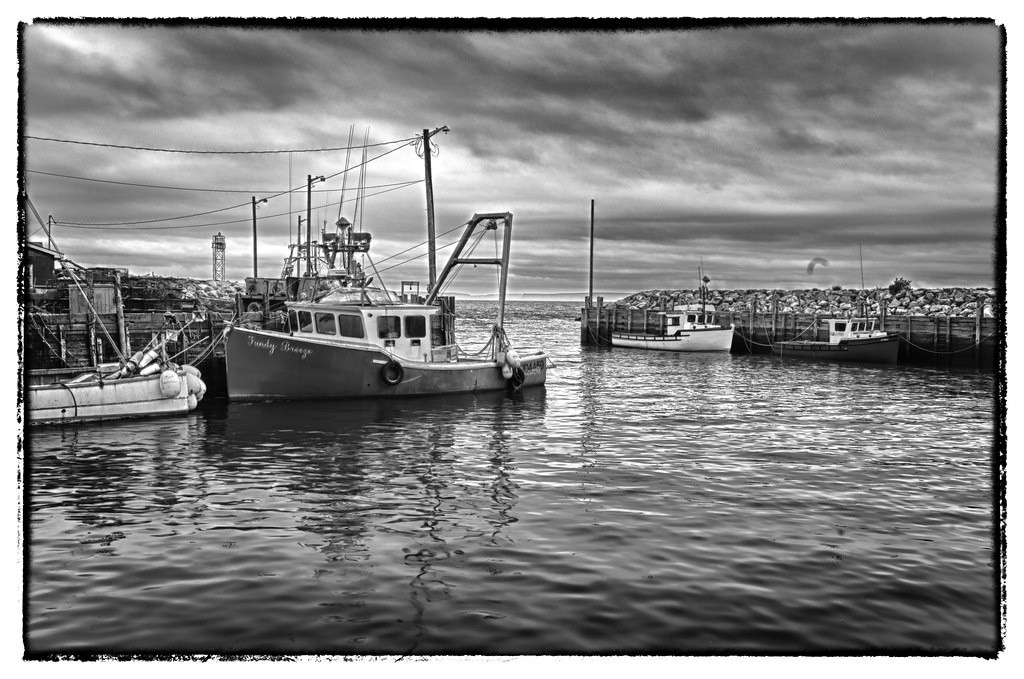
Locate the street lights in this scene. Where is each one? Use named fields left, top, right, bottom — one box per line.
left=250, top=196, right=269, bottom=278
left=307, top=174, right=325, bottom=275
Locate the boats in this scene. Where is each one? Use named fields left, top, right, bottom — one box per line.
left=223, top=129, right=548, bottom=400
left=611, top=257, right=735, bottom=352
left=26, top=314, right=209, bottom=428
left=772, top=242, right=901, bottom=365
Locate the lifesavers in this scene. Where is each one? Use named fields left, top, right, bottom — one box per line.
left=247, top=302, right=263, bottom=321
left=507, top=368, right=525, bottom=390
left=382, top=360, right=404, bottom=386
